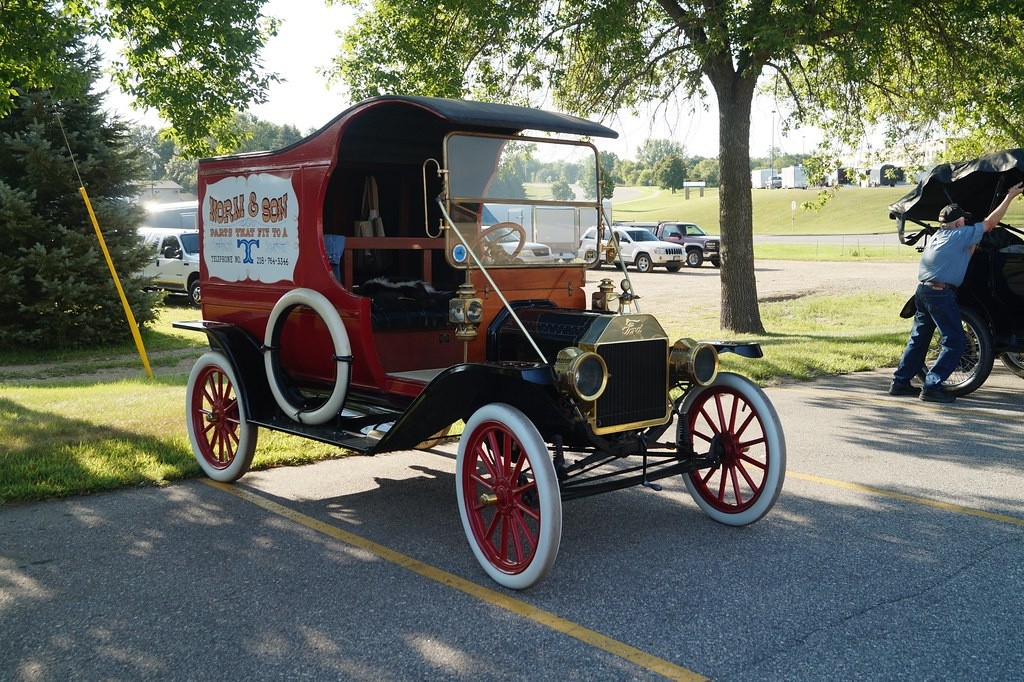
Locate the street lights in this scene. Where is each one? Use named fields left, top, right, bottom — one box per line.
left=770, top=108, right=776, bottom=191
left=803, top=136, right=807, bottom=168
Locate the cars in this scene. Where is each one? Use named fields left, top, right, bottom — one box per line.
left=478, top=225, right=552, bottom=266
left=130, top=227, right=205, bottom=309
left=173, top=92, right=788, bottom=592
left=766, top=176, right=781, bottom=189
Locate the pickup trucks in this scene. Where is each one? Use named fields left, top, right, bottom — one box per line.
left=614, top=219, right=722, bottom=269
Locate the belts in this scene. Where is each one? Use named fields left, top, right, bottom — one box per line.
left=920, top=280, right=952, bottom=288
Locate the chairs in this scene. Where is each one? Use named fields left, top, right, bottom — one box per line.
left=984, top=227, right=1024, bottom=299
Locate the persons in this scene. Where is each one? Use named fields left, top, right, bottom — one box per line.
left=889, top=182, right=1024, bottom=402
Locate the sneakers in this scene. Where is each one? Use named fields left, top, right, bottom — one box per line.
left=889, top=379, right=922, bottom=396
left=919, top=382, right=956, bottom=403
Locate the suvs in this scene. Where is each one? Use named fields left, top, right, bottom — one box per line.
left=576, top=225, right=689, bottom=273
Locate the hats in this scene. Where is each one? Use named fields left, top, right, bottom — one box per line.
left=938, top=203, right=971, bottom=224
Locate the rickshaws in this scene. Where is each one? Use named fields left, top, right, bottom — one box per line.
left=887, top=145, right=1024, bottom=397
